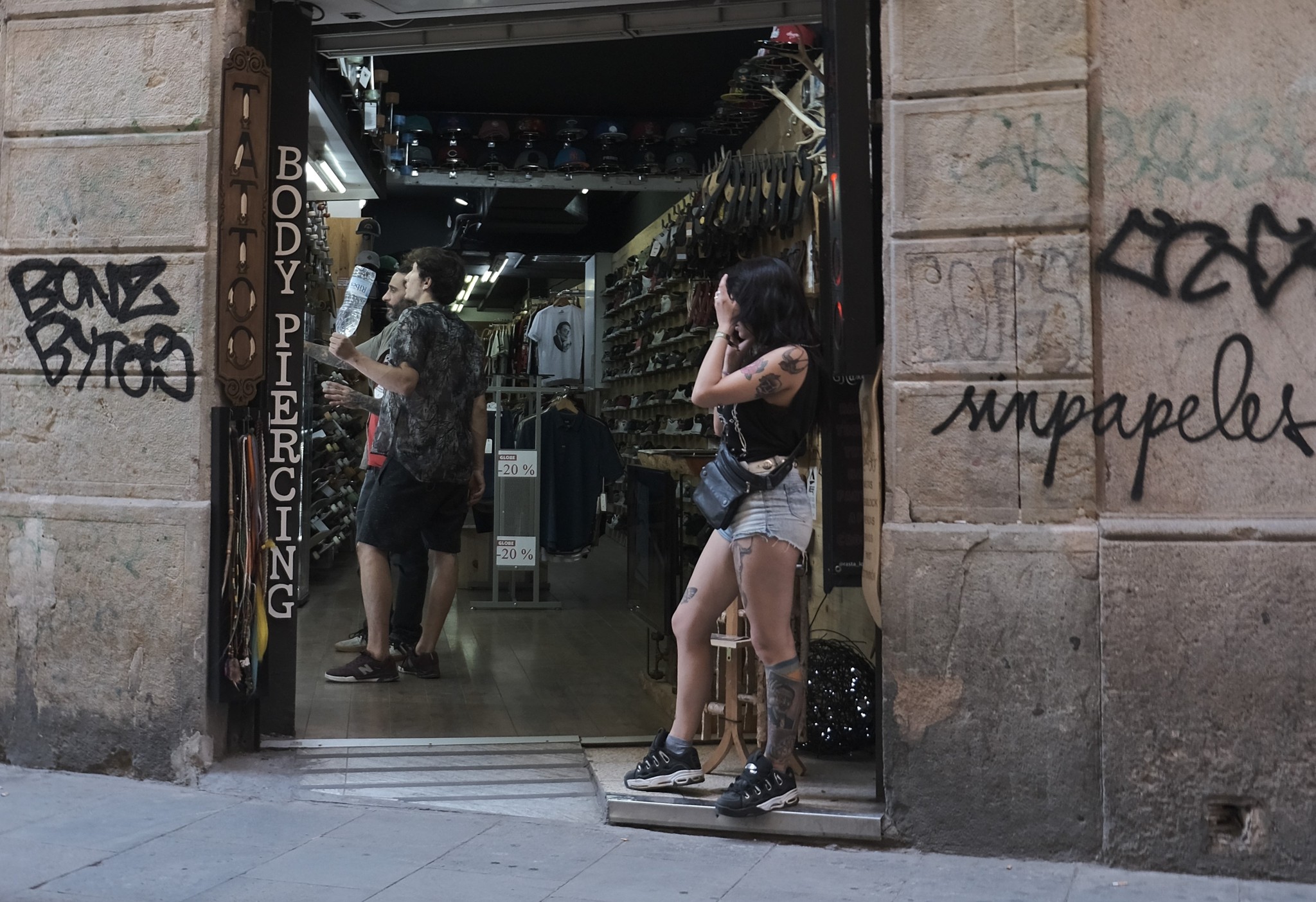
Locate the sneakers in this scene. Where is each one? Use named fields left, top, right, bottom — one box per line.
left=334, top=628, right=368, bottom=652
left=602, top=244, right=718, bottom=458
left=324, top=648, right=400, bottom=682
left=400, top=647, right=440, bottom=678
left=713, top=749, right=800, bottom=815
left=388, top=637, right=419, bottom=658
left=623, top=729, right=706, bottom=789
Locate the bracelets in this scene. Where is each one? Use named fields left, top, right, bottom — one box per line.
left=714, top=331, right=731, bottom=341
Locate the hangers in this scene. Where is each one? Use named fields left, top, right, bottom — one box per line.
left=483, top=289, right=571, bottom=341
left=550, top=387, right=579, bottom=414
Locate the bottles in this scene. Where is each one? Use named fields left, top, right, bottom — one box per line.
left=336, top=266, right=377, bottom=336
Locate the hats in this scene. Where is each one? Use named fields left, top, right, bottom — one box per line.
left=355, top=219, right=399, bottom=300
left=697, top=24, right=814, bottom=137
left=391, top=115, right=697, bottom=172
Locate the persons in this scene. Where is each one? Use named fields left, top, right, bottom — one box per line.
left=625, top=256, right=819, bottom=819
left=304, top=246, right=484, bottom=683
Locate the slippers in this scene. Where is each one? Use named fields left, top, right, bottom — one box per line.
left=691, top=153, right=812, bottom=274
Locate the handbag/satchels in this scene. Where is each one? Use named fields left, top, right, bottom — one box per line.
left=692, top=446, right=759, bottom=530
